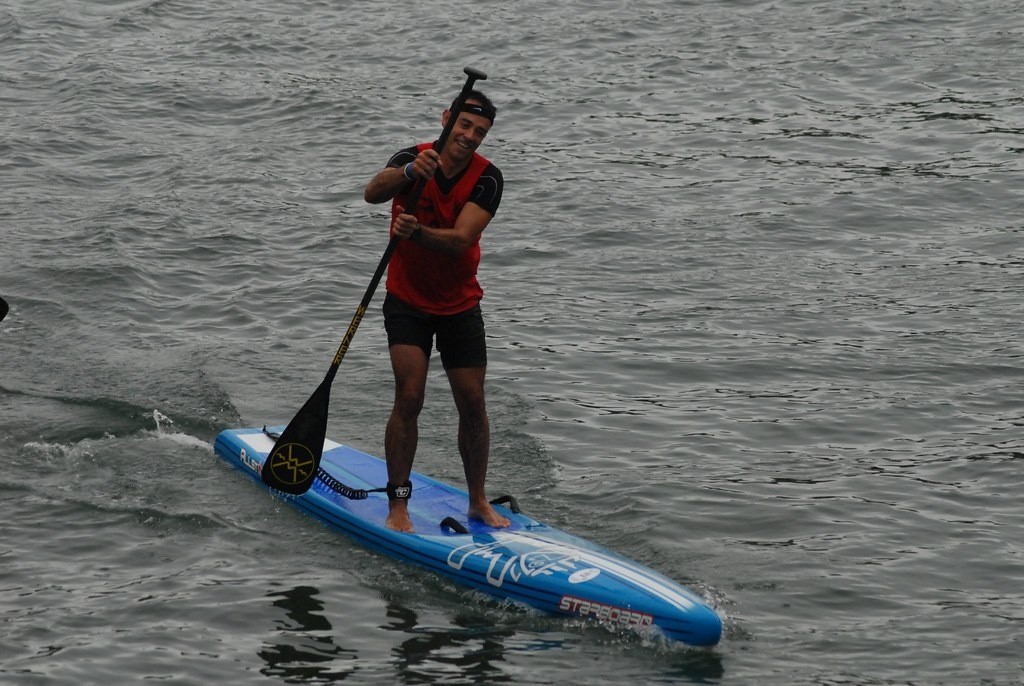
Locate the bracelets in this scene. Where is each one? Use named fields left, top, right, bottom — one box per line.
left=404, top=163, right=415, bottom=180
left=410, top=224, right=421, bottom=241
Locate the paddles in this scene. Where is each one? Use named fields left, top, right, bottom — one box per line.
left=261, top=64, right=486, bottom=493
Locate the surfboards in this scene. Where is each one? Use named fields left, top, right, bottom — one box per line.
left=209, top=421, right=726, bottom=651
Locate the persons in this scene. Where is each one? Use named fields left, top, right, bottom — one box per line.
left=364, top=90, right=512, bottom=533
